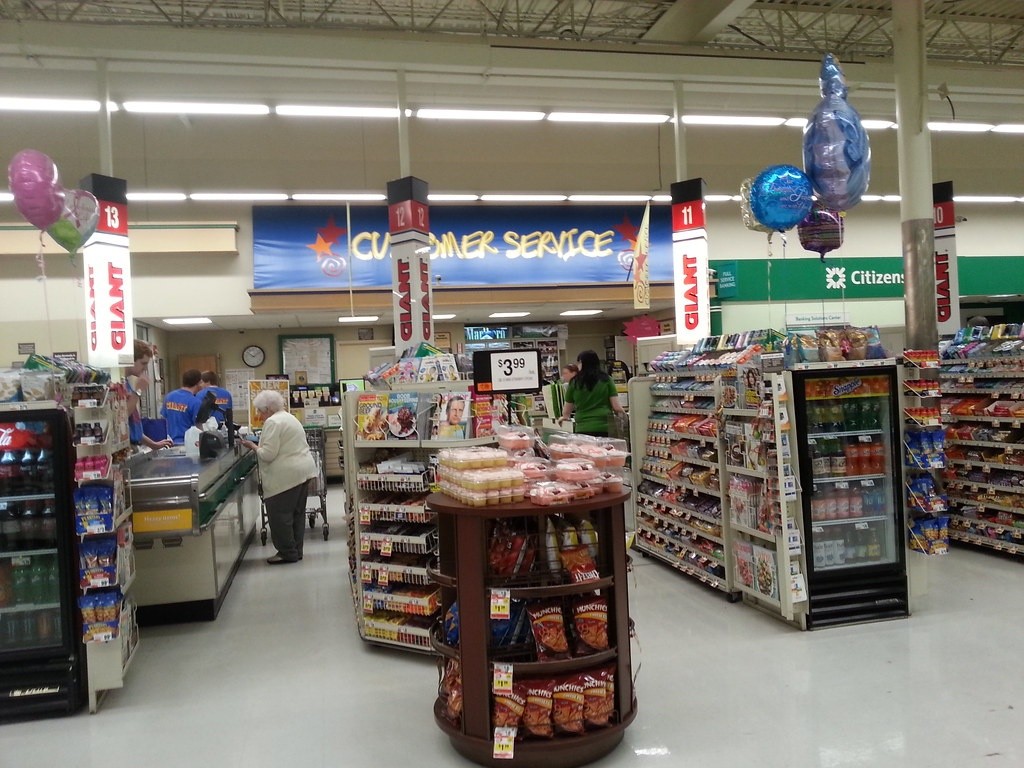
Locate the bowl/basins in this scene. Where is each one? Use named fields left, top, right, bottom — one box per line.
left=435, top=425, right=632, bottom=508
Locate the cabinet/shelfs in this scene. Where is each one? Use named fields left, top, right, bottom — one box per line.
left=71, top=382, right=141, bottom=712
left=341, top=333, right=1024, bottom=768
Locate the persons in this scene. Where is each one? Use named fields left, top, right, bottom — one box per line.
left=160, top=370, right=203, bottom=446
left=743, top=368, right=761, bottom=392
left=563, top=364, right=579, bottom=382
left=969, top=316, right=989, bottom=327
left=432, top=393, right=464, bottom=440
left=126, top=339, right=173, bottom=450
left=558, top=350, right=628, bottom=438
left=241, top=390, right=318, bottom=564
left=196, top=371, right=233, bottom=421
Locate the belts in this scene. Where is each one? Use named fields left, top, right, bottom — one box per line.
left=131, top=440, right=143, bottom=446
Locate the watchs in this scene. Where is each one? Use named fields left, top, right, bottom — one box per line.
left=133, top=391, right=142, bottom=397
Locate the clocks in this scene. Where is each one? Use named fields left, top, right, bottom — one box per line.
left=242, top=345, right=265, bottom=367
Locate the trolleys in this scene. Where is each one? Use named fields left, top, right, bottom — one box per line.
left=251, top=425, right=329, bottom=547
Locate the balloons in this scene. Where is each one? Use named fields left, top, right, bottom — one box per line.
left=9, top=148, right=66, bottom=231
left=741, top=52, right=871, bottom=257
left=47, top=189, right=100, bottom=254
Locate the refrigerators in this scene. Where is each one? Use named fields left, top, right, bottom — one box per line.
left=777, top=358, right=911, bottom=630
left=0, top=400, right=86, bottom=721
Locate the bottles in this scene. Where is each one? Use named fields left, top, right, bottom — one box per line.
left=74, top=422, right=103, bottom=445
left=0, top=446, right=62, bottom=646
left=75, top=455, right=108, bottom=481
left=184, top=426, right=203, bottom=457
left=805, top=400, right=888, bottom=568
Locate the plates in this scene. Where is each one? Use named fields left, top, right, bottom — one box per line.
left=388, top=415, right=417, bottom=437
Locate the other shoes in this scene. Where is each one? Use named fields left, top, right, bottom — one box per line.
left=265, top=554, right=303, bottom=564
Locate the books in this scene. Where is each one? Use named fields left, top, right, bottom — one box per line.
left=366, top=342, right=461, bottom=386
left=430, top=392, right=472, bottom=440
left=357, top=392, right=418, bottom=440
